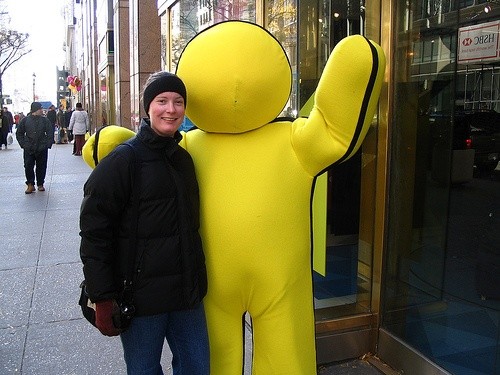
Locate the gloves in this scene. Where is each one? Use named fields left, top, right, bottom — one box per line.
left=95, top=299, right=121, bottom=336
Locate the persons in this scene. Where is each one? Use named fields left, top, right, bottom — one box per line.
left=77, top=71, right=211, bottom=375
left=47, top=101, right=90, bottom=157
left=0, top=106, right=25, bottom=151
left=15, top=101, right=53, bottom=194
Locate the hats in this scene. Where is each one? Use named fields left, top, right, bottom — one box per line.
left=30, top=102, right=43, bottom=112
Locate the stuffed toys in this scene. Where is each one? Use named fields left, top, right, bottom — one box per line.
left=82, top=19, right=386, bottom=375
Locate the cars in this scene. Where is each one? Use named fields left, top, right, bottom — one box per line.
left=421, top=106, right=499, bottom=183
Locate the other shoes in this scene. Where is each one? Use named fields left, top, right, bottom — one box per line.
left=38, top=184, right=46, bottom=191
left=24, top=183, right=36, bottom=193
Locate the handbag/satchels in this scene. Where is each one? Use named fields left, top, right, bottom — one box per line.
left=76, top=142, right=143, bottom=337
left=7, top=132, right=14, bottom=145
